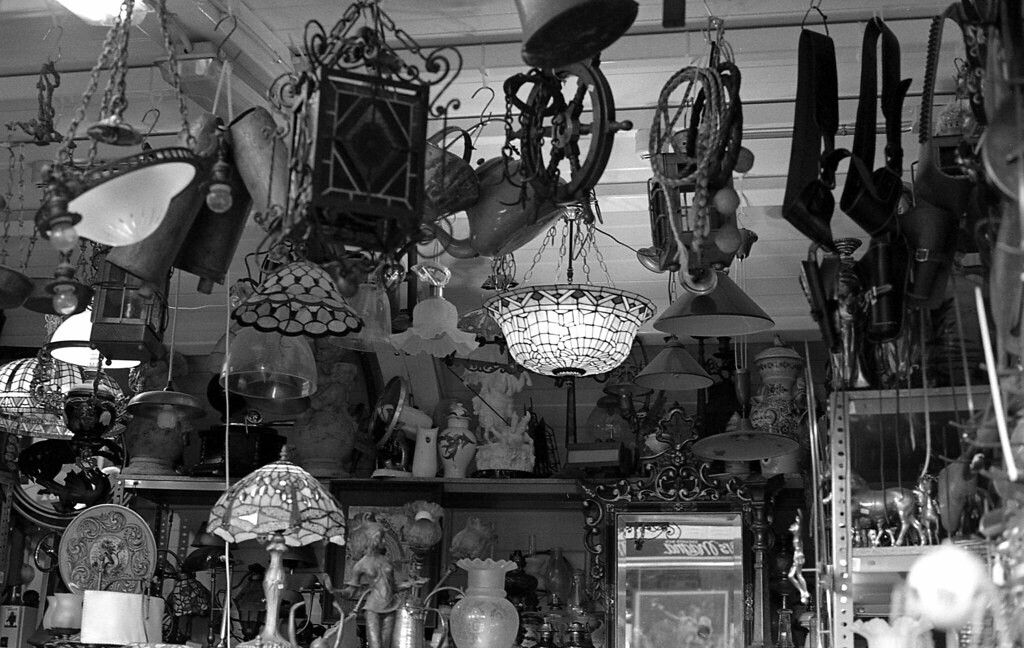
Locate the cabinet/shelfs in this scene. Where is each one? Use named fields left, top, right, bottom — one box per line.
left=827, top=386, right=993, bottom=648
left=333, top=471, right=782, bottom=632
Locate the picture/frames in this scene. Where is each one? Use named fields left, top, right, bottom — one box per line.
left=578, top=409, right=774, bottom=648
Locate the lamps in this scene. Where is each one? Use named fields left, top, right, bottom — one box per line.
left=633, top=273, right=715, bottom=392
left=391, top=220, right=479, bottom=359
left=482, top=204, right=655, bottom=386
left=206, top=446, right=346, bottom=648
left=45, top=240, right=141, bottom=370
left=37, top=0, right=197, bottom=245
left=230, top=70, right=363, bottom=340
left=653, top=267, right=777, bottom=337
left=125, top=268, right=209, bottom=429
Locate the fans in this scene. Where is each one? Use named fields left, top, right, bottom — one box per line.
left=366, top=374, right=432, bottom=478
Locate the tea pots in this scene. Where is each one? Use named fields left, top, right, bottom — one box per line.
left=421, top=156, right=565, bottom=261
